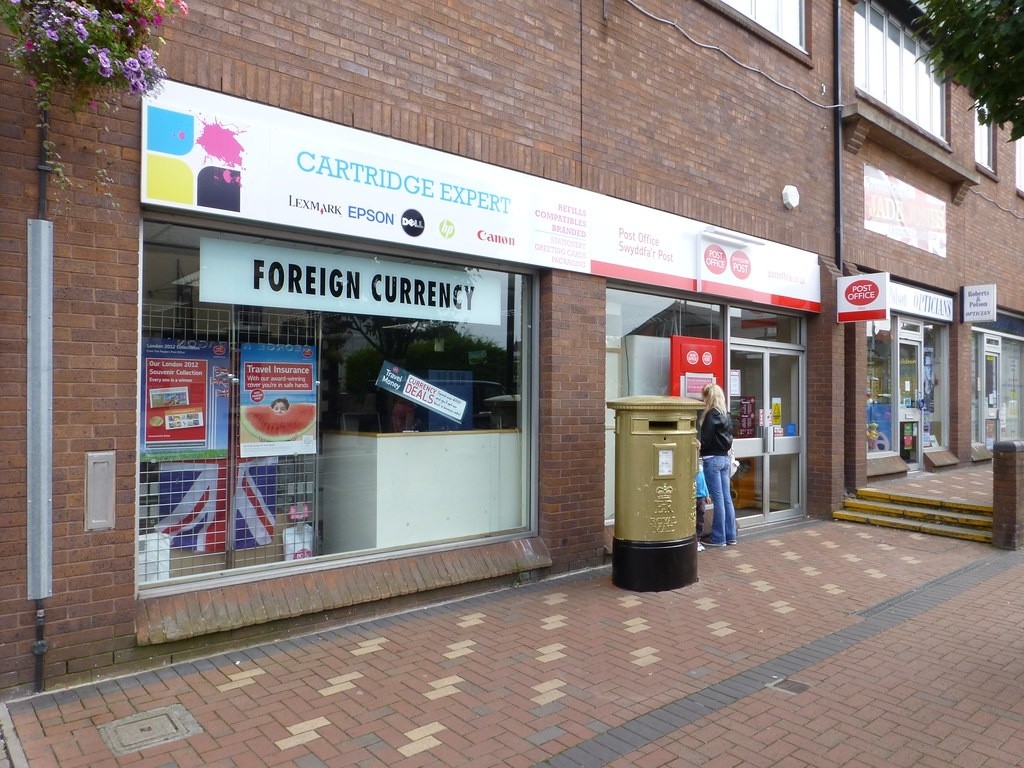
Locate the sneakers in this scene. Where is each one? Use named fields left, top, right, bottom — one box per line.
left=699, top=534, right=738, bottom=547
left=696, top=542, right=706, bottom=552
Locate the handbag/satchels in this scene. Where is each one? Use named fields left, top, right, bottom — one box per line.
left=727, top=441, right=740, bottom=477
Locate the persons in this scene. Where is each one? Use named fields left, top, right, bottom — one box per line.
left=696, top=460, right=711, bottom=552
left=699, top=383, right=738, bottom=546
left=259, top=397, right=297, bottom=443
